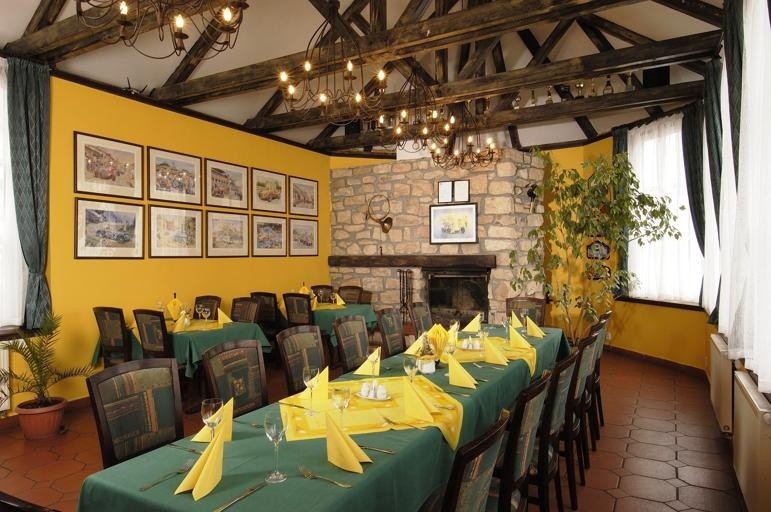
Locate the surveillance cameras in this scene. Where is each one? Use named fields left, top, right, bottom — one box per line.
left=527, top=184, right=538, bottom=198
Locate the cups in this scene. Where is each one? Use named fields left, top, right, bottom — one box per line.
left=196, top=303, right=206, bottom=321
left=478, top=324, right=490, bottom=357
left=331, top=293, right=336, bottom=304
left=502, top=317, right=512, bottom=340
left=202, top=307, right=211, bottom=321
left=318, top=289, right=325, bottom=303
left=333, top=386, right=350, bottom=432
left=303, top=365, right=320, bottom=416
left=444, top=341, right=457, bottom=356
left=264, top=410, right=287, bottom=482
left=521, top=308, right=530, bottom=333
left=450, top=319, right=460, bottom=331
left=404, top=355, right=420, bottom=382
left=489, top=305, right=498, bottom=329
left=475, top=311, right=485, bottom=323
left=201, top=397, right=224, bottom=440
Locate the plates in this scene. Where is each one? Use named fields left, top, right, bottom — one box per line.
left=459, top=347, right=485, bottom=351
left=356, top=391, right=392, bottom=400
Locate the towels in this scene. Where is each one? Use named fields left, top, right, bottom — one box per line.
left=190, top=397, right=233, bottom=442
left=166, top=319, right=223, bottom=331
left=483, top=334, right=511, bottom=366
left=402, top=375, right=441, bottom=421
left=439, top=336, right=536, bottom=378
left=175, top=422, right=224, bottom=501
left=300, top=367, right=328, bottom=401
left=449, top=353, right=479, bottom=390
left=167, top=313, right=185, bottom=333
left=218, top=308, right=233, bottom=324
left=512, top=311, right=522, bottom=328
left=279, top=374, right=463, bottom=451
left=326, top=411, right=373, bottom=473
left=353, top=345, right=381, bottom=376
left=462, top=313, right=481, bottom=332
left=526, top=316, right=546, bottom=338
left=336, top=293, right=345, bottom=305
left=404, top=332, right=431, bottom=356
left=509, top=325, right=531, bottom=348
left=316, top=302, right=345, bottom=310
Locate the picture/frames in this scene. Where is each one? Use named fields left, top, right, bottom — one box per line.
left=148, top=204, right=203, bottom=258
left=204, top=157, right=249, bottom=210
left=206, top=210, right=250, bottom=257
left=73, top=131, right=144, bottom=199
left=429, top=202, right=478, bottom=245
left=75, top=197, right=146, bottom=259
left=251, top=167, right=287, bottom=214
left=252, top=214, right=287, bottom=256
left=289, top=175, right=318, bottom=217
left=289, top=218, right=319, bottom=257
left=147, top=146, right=202, bottom=205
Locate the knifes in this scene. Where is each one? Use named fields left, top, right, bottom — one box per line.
left=214, top=482, right=267, bottom=512
left=359, top=445, right=396, bottom=454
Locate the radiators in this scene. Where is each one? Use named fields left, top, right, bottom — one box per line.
left=710, top=334, right=732, bottom=432
left=734, top=370, right=771, bottom=512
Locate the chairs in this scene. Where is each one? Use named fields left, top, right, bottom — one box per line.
left=407, top=303, right=432, bottom=340
left=338, top=286, right=363, bottom=304
left=85, top=359, right=184, bottom=469
left=282, top=294, right=336, bottom=369
left=276, top=325, right=326, bottom=397
left=133, top=309, right=203, bottom=394
left=332, top=315, right=370, bottom=374
left=422, top=408, right=511, bottom=512
left=597, top=309, right=612, bottom=439
left=505, top=297, right=546, bottom=328
left=374, top=308, right=407, bottom=357
left=250, top=292, right=288, bottom=369
left=203, top=339, right=267, bottom=420
left=569, top=336, right=600, bottom=486
left=311, top=285, right=334, bottom=303
left=231, top=297, right=261, bottom=324
left=194, top=296, right=222, bottom=320
left=92, top=308, right=131, bottom=369
left=531, top=350, right=580, bottom=512
left=492, top=371, right=551, bottom=511
left=591, top=322, right=611, bottom=450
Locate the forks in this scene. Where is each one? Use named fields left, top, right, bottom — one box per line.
left=298, top=463, right=352, bottom=488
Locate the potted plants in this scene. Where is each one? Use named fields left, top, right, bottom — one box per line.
left=453, top=180, right=470, bottom=204
left=0, top=311, right=98, bottom=439
left=437, top=181, right=453, bottom=203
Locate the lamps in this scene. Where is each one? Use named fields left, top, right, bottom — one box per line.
left=426, top=100, right=495, bottom=170
left=279, top=0, right=390, bottom=124
left=385, top=52, right=455, bottom=153
left=76, top=0, right=249, bottom=63
